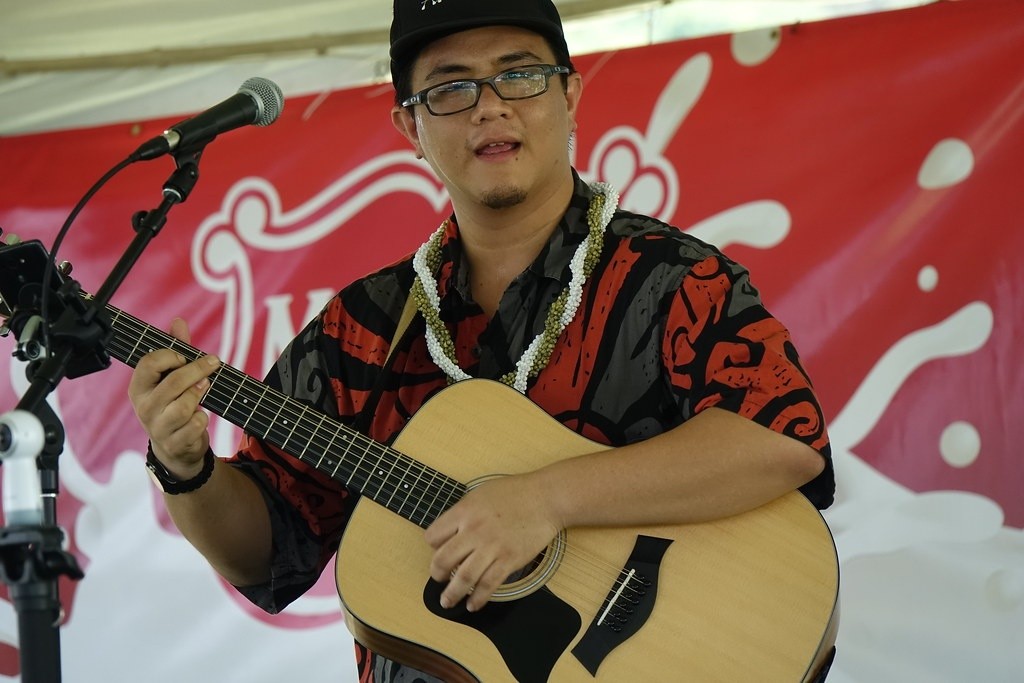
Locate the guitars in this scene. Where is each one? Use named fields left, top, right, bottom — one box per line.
left=0, top=230, right=844, bottom=683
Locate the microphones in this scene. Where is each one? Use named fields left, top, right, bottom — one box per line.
left=129, top=77, right=285, bottom=161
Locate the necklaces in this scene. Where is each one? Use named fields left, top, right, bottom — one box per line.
left=410, top=181, right=620, bottom=394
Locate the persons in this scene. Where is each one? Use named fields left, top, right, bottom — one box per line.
left=124, top=0, right=843, bottom=683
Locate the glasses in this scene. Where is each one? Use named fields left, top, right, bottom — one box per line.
left=401, top=63, right=571, bottom=118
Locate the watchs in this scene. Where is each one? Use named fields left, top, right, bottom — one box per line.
left=143, top=435, right=216, bottom=496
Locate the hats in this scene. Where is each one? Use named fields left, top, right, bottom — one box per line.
left=388, top=0, right=570, bottom=89
